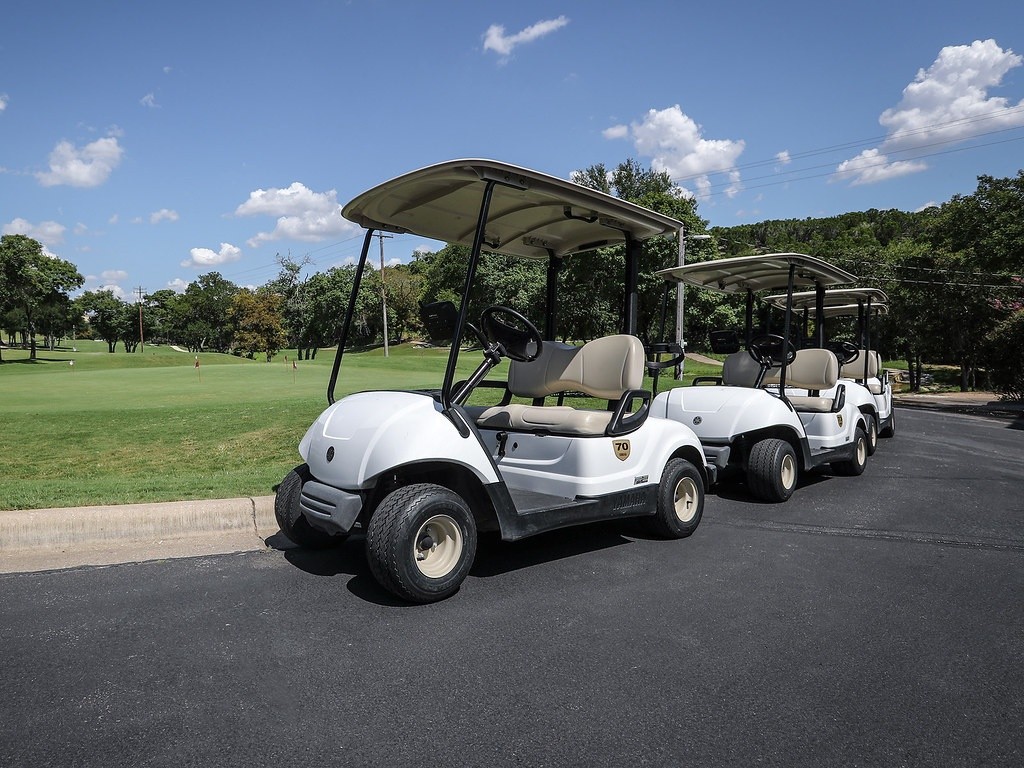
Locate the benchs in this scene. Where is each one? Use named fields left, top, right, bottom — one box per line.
left=839, top=350, right=883, bottom=395
left=721, top=348, right=839, bottom=413
left=461, top=332, right=647, bottom=441
